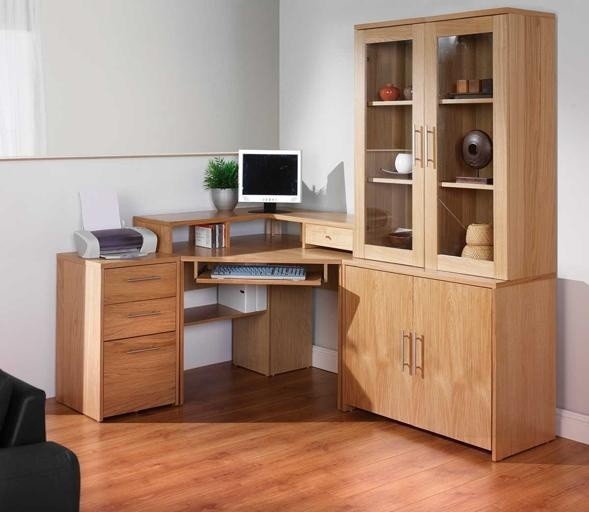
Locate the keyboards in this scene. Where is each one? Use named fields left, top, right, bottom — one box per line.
left=211, top=264, right=307, bottom=280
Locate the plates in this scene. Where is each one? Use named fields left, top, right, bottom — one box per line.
left=379, top=169, right=416, bottom=178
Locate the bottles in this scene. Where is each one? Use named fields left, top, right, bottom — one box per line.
left=394, top=152, right=412, bottom=172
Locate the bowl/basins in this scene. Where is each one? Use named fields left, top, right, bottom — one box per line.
left=389, top=232, right=411, bottom=248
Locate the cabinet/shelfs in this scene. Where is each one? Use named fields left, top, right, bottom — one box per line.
left=129, top=206, right=354, bottom=254
left=56, top=250, right=181, bottom=423
left=353, top=8, right=558, bottom=280
left=341, top=256, right=556, bottom=462
left=180, top=234, right=342, bottom=416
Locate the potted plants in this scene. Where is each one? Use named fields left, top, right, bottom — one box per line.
left=202, top=152, right=239, bottom=211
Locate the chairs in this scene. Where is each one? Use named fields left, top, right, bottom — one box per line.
left=0, top=368, right=80, bottom=512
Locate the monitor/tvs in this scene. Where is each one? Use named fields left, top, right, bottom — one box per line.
left=239, top=150, right=302, bottom=213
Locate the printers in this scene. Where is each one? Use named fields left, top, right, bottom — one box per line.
left=74, top=219, right=158, bottom=260
